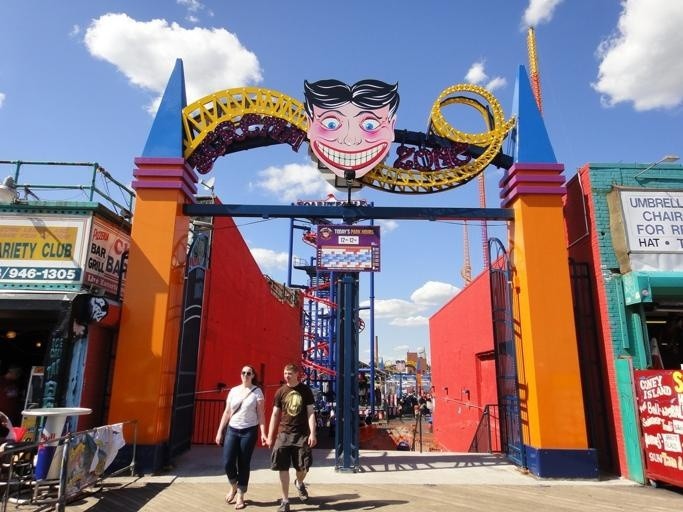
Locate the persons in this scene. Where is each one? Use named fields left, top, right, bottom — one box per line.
left=215, top=366, right=267, bottom=509
left=400, top=391, right=432, bottom=420
left=302, top=79, right=400, bottom=179
left=267, top=364, right=319, bottom=512
left=1, top=364, right=23, bottom=430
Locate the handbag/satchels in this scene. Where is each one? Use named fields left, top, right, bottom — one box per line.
left=218, top=423, right=227, bottom=447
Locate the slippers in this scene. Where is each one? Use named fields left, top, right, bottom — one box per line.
left=234, top=496, right=245, bottom=510
left=223, top=488, right=237, bottom=503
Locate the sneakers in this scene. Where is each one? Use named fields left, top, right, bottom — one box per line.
left=294, top=478, right=309, bottom=502
left=276, top=499, right=290, bottom=512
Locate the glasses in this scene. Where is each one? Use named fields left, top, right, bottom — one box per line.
left=240, top=371, right=253, bottom=376
